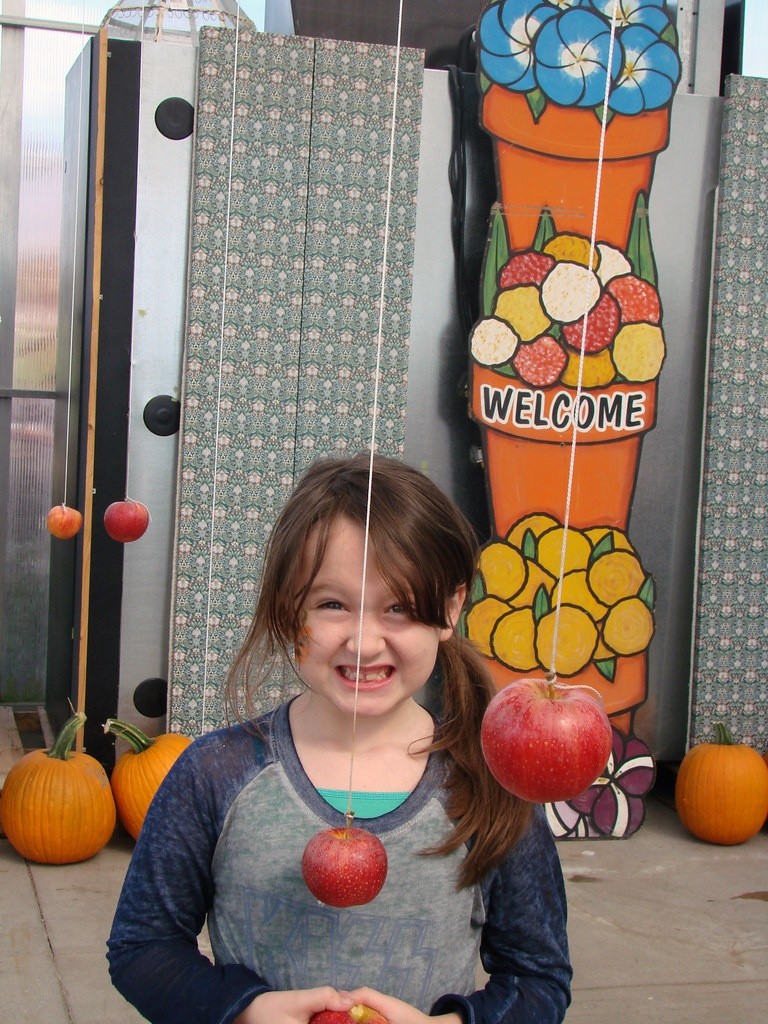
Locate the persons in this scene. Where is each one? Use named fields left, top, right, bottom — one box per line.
left=106, top=455, right=574, bottom=1023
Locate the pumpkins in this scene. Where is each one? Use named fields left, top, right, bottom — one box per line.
left=0, top=712, right=117, bottom=864
left=103, top=718, right=193, bottom=842
left=674, top=723, right=768, bottom=845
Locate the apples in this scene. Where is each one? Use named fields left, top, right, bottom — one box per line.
left=479, top=671, right=612, bottom=802
left=104, top=499, right=149, bottom=542
left=302, top=811, right=387, bottom=907
left=47, top=505, right=83, bottom=539
left=307, top=1002, right=390, bottom=1024
